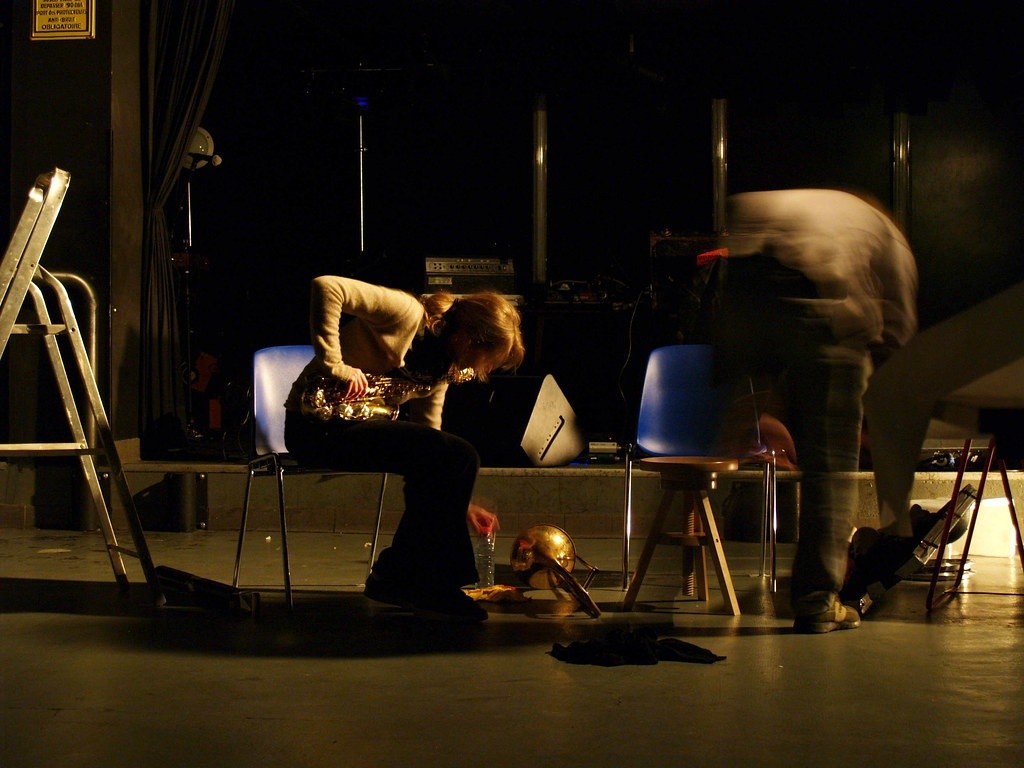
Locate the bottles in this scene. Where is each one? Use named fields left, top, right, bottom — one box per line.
left=476, top=527, right=495, bottom=586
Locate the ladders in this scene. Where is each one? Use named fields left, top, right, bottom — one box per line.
left=0, top=163, right=168, bottom=611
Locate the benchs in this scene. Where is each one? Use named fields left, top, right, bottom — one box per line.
left=98, top=462, right=1024, bottom=543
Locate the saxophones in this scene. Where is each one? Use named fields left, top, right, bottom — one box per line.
left=299, top=362, right=478, bottom=424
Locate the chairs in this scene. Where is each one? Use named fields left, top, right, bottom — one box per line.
left=624, top=344, right=779, bottom=593
left=232, top=345, right=393, bottom=614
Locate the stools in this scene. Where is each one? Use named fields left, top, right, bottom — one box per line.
left=616, top=456, right=741, bottom=614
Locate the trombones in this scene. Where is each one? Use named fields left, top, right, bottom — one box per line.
left=509, top=523, right=601, bottom=619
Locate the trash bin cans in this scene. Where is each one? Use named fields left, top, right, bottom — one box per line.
left=162, top=471, right=200, bottom=534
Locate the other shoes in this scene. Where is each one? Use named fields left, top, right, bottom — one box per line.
left=364, top=571, right=416, bottom=610
left=793, top=604, right=861, bottom=634
left=414, top=587, right=489, bottom=621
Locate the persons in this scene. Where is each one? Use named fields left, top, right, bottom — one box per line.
left=664, top=189, right=916, bottom=633
left=285, top=276, right=525, bottom=624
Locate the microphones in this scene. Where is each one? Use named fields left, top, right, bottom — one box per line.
left=188, top=152, right=221, bottom=165
